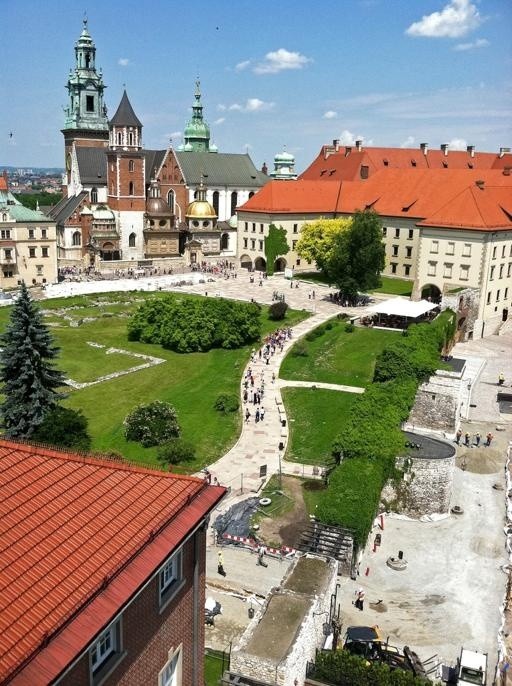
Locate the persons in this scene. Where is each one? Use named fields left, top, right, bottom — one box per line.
left=213, top=476, right=220, bottom=486
left=254, top=542, right=269, bottom=568
left=456, top=428, right=463, bottom=446
left=328, top=291, right=366, bottom=307
left=484, top=431, right=495, bottom=447
left=464, top=432, right=471, bottom=446
left=204, top=471, right=212, bottom=484
left=59, top=259, right=269, bottom=287
left=270, top=279, right=317, bottom=303
left=473, top=431, right=483, bottom=446
left=238, top=325, right=294, bottom=424
left=354, top=587, right=366, bottom=611
left=498, top=372, right=506, bottom=385
left=217, top=551, right=227, bottom=577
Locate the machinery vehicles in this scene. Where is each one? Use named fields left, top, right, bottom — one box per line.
left=337, top=623, right=442, bottom=679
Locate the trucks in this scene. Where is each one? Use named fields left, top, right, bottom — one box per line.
left=453, top=646, right=490, bottom=686
